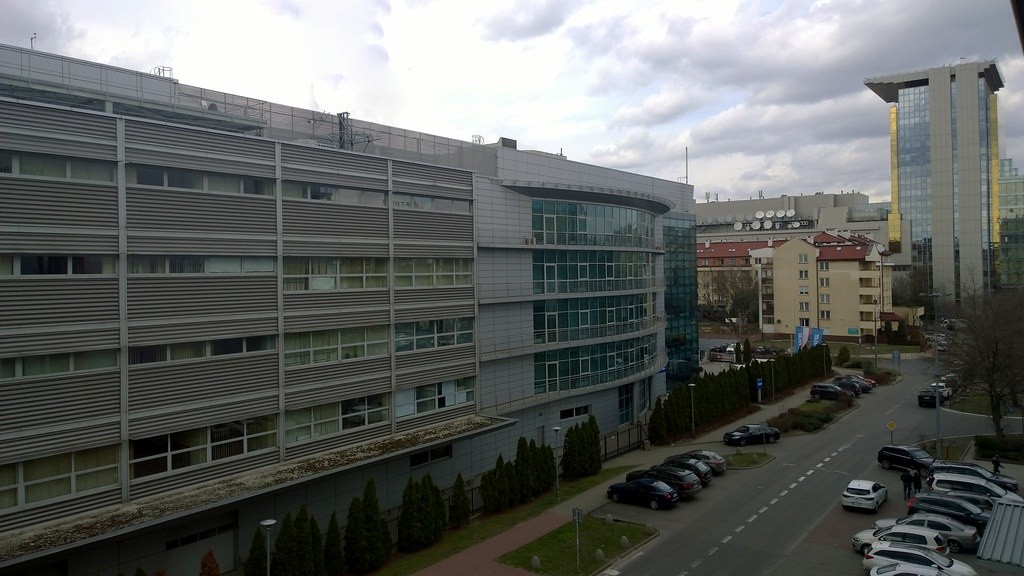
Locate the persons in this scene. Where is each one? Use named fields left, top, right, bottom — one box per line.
left=992, top=454, right=1005, bottom=473
left=901, top=465, right=936, bottom=500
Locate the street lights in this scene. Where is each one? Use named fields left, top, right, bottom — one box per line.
left=552, top=427, right=563, bottom=502
left=688, top=383, right=696, bottom=433
left=260, top=519, right=276, bottom=576
left=770, top=359, right=775, bottom=400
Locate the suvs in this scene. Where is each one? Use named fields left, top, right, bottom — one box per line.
left=651, top=458, right=713, bottom=484
left=931, top=382, right=952, bottom=399
left=810, top=383, right=850, bottom=401
left=906, top=492, right=990, bottom=536
left=832, top=376, right=872, bottom=393
left=926, top=461, right=1019, bottom=494
left=926, top=471, right=1024, bottom=505
left=851, top=525, right=950, bottom=559
left=842, top=478, right=888, bottom=514
left=917, top=388, right=945, bottom=408
left=877, top=443, right=936, bottom=479
left=626, top=465, right=700, bottom=499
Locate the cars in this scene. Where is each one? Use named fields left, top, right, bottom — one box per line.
left=726, top=344, right=784, bottom=356
left=870, top=563, right=941, bottom=576
left=847, top=374, right=876, bottom=387
left=665, top=450, right=727, bottom=472
left=864, top=541, right=977, bottom=576
left=606, top=479, right=680, bottom=510
left=834, top=382, right=861, bottom=398
left=872, top=512, right=979, bottom=553
left=723, top=424, right=780, bottom=447
